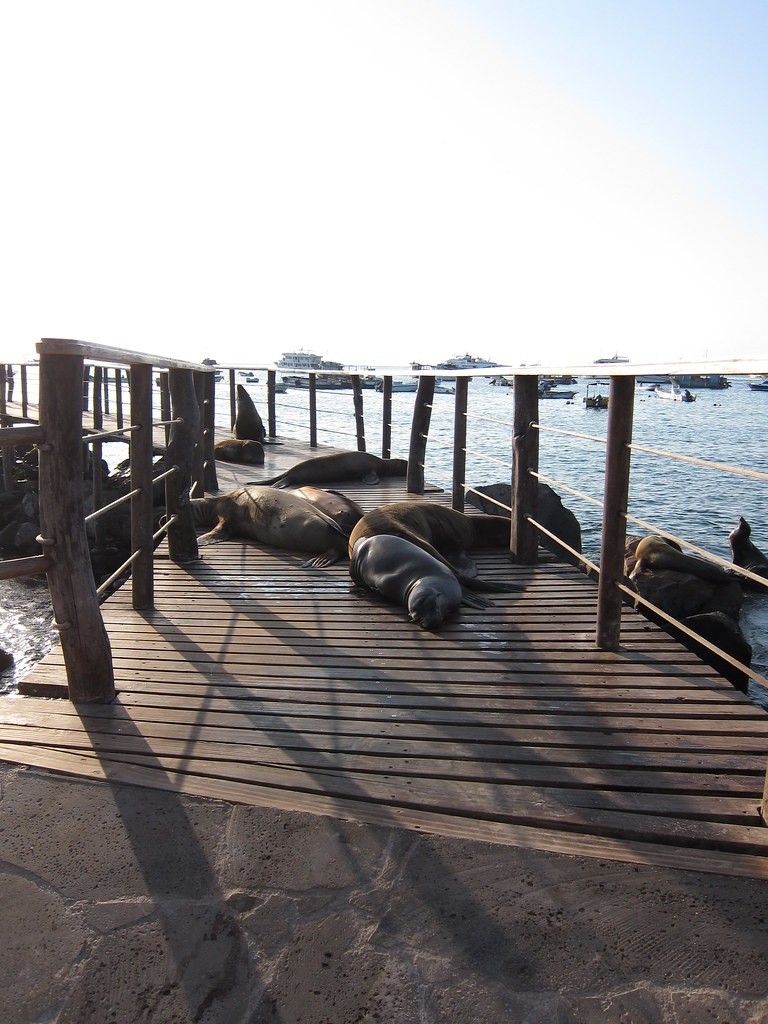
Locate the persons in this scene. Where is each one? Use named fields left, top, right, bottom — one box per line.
left=685, top=389, right=690, bottom=397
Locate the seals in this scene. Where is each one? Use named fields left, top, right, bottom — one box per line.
left=243, top=451, right=408, bottom=489
left=629, top=516, right=768, bottom=581
left=232, top=384, right=285, bottom=446
left=214, top=439, right=265, bottom=465
left=190, top=484, right=528, bottom=635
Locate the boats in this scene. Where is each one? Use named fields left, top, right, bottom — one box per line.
left=315, top=360, right=345, bottom=371
left=246, top=377, right=258, bottom=383
left=88, top=374, right=130, bottom=383
left=582, top=382, right=612, bottom=410
left=592, top=352, right=632, bottom=364
left=485, top=374, right=579, bottom=400
left=436, top=351, right=513, bottom=371
left=272, top=371, right=456, bottom=394
left=240, top=372, right=254, bottom=378
left=655, top=377, right=697, bottom=403
left=410, top=360, right=474, bottom=382
left=746, top=378, right=768, bottom=391
left=155, top=358, right=224, bottom=386
left=274, top=345, right=325, bottom=369
left=636, top=374, right=733, bottom=390
